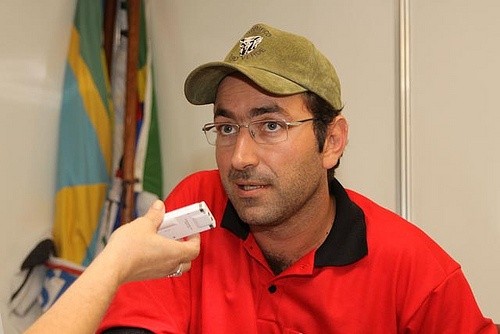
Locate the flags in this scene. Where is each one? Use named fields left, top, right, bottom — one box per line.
left=38, top=0, right=164, bottom=316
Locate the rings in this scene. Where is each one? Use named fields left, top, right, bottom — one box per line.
left=168, top=268, right=183, bottom=278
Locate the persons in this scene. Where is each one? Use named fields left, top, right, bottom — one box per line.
left=96, top=23, right=500, bottom=334
left=22, top=199, right=201, bottom=334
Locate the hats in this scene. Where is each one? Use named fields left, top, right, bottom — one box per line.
left=184, top=23, right=345, bottom=114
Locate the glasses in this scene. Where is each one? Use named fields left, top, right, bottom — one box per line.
left=202, top=117, right=317, bottom=147
